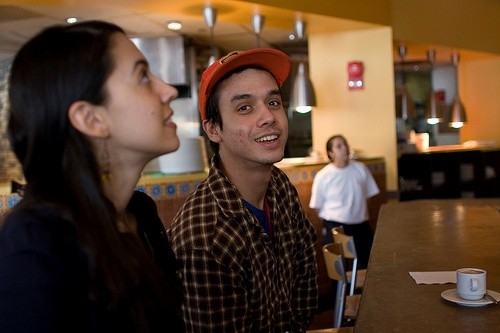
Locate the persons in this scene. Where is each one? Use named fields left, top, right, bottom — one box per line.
left=1, top=22, right=187, bottom=333
left=307, top=136, right=380, bottom=270
left=166, top=48, right=318, bottom=333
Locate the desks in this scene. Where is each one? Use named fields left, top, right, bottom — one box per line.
left=354, top=198, right=500, bottom=333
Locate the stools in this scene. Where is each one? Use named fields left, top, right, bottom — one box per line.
left=320, top=241, right=364, bottom=328
left=330, top=224, right=366, bottom=294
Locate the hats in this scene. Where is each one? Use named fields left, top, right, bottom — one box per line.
left=198, top=48, right=292, bottom=120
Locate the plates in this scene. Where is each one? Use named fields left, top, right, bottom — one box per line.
left=157, top=138, right=205, bottom=175
left=440, top=289, right=494, bottom=307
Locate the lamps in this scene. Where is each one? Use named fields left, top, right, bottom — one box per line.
left=251, top=13, right=266, bottom=46
left=397, top=45, right=415, bottom=120
left=288, top=17, right=318, bottom=113
left=203, top=6, right=218, bottom=67
left=447, top=50, right=467, bottom=128
left=424, top=50, right=445, bottom=125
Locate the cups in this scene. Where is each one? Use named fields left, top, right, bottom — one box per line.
left=456, top=268, right=486, bottom=301
